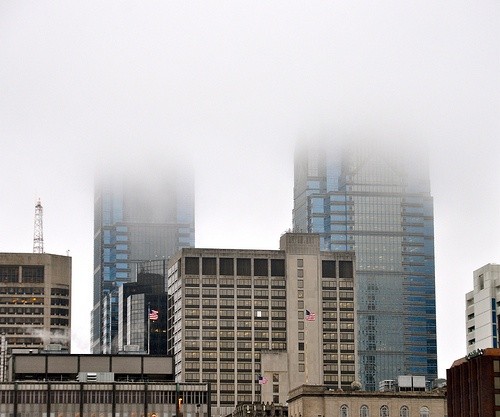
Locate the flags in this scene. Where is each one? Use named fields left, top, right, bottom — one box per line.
left=305, top=310, right=316, bottom=321
left=149, top=310, right=159, bottom=320
left=259, top=376, right=269, bottom=384
